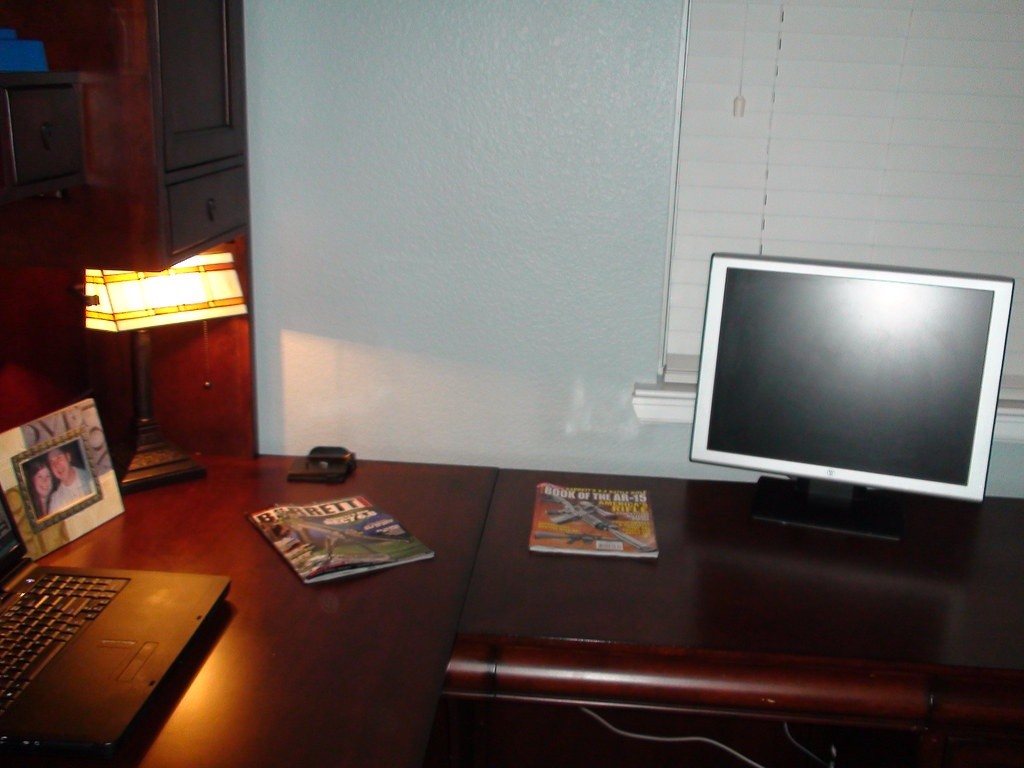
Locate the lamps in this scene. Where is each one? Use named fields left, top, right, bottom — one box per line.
left=86, top=243, right=248, bottom=488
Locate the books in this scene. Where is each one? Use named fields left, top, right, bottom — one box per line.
left=528, top=483, right=659, bottom=559
left=247, top=495, right=435, bottom=585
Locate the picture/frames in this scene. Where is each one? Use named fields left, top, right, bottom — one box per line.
left=0, top=399, right=126, bottom=563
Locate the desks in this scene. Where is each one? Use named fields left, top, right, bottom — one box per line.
left=0, top=444, right=1024, bottom=768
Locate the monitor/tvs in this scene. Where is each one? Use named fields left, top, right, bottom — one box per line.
left=688, top=254, right=1015, bottom=543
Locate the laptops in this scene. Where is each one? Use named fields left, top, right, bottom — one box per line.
left=0, top=484, right=231, bottom=755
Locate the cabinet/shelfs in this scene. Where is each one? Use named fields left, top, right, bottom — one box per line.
left=0, top=0, right=249, bottom=274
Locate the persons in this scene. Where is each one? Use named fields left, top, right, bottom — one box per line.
left=24, top=447, right=93, bottom=519
